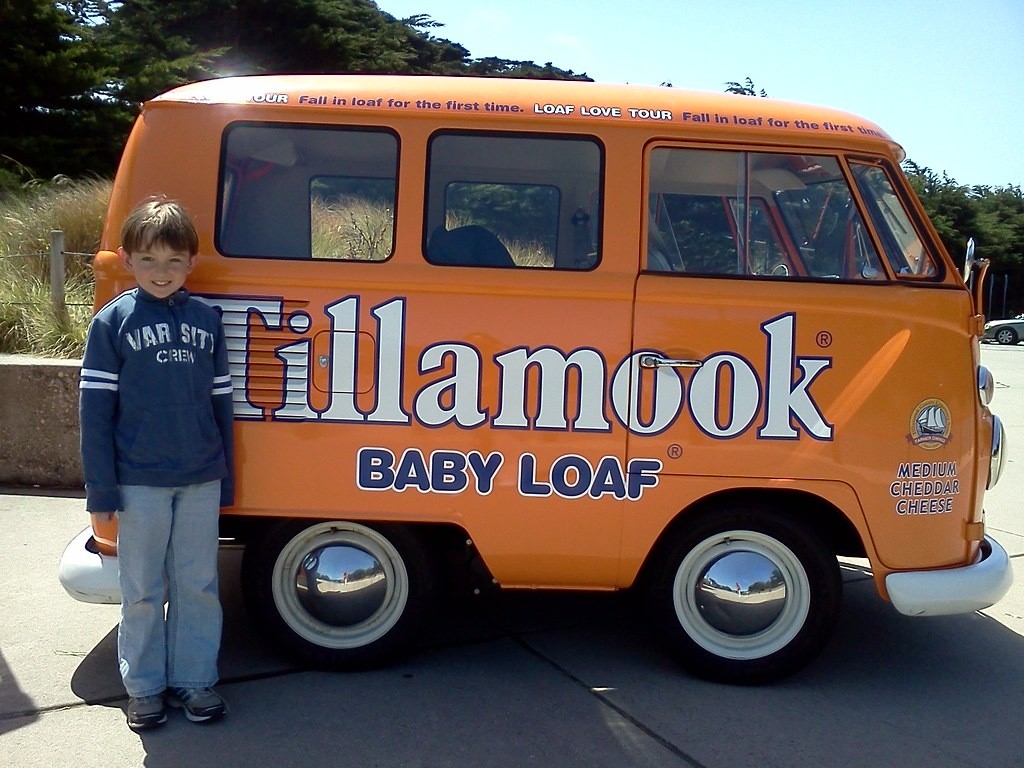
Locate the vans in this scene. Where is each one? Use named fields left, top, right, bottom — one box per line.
left=90, top=75, right=1017, bottom=674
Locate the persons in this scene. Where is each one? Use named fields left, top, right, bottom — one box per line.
left=79, top=194, right=235, bottom=730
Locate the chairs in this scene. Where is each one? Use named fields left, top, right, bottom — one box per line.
left=431, top=226, right=516, bottom=266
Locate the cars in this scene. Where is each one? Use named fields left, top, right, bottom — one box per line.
left=983, top=314, right=1024, bottom=345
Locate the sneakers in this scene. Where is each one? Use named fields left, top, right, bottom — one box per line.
left=165, top=683, right=228, bottom=724
left=127, top=692, right=167, bottom=730
